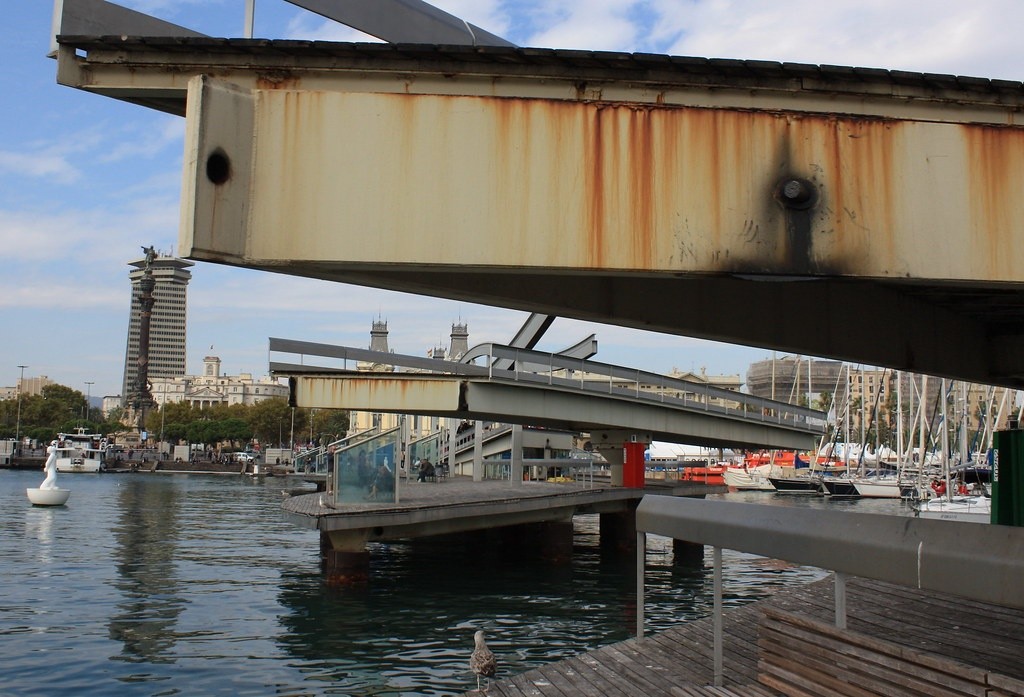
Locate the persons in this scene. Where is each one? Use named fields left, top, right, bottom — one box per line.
left=277, top=456, right=436, bottom=501
left=41, top=446, right=59, bottom=490
left=115, top=450, right=232, bottom=470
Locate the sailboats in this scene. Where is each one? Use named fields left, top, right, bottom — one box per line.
left=681, top=351, right=1024, bottom=514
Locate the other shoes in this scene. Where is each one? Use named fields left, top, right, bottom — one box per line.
left=363, top=496, right=377, bottom=501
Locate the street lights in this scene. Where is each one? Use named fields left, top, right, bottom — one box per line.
left=15, top=365, right=29, bottom=441
left=85, top=381, right=94, bottom=420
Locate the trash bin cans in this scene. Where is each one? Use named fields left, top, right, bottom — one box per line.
left=991, top=426, right=1024, bottom=525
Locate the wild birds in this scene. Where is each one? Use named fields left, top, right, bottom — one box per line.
left=470, top=630, right=497, bottom=691
left=319, top=495, right=336, bottom=511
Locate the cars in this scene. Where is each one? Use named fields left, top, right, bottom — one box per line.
left=234, top=452, right=253, bottom=462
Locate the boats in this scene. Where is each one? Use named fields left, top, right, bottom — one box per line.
left=50, top=423, right=107, bottom=472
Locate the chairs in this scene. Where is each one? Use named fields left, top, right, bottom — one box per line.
left=427, top=466, right=436, bottom=482
left=435, top=465, right=445, bottom=480
left=931, top=481, right=947, bottom=498
left=442, top=463, right=450, bottom=478
